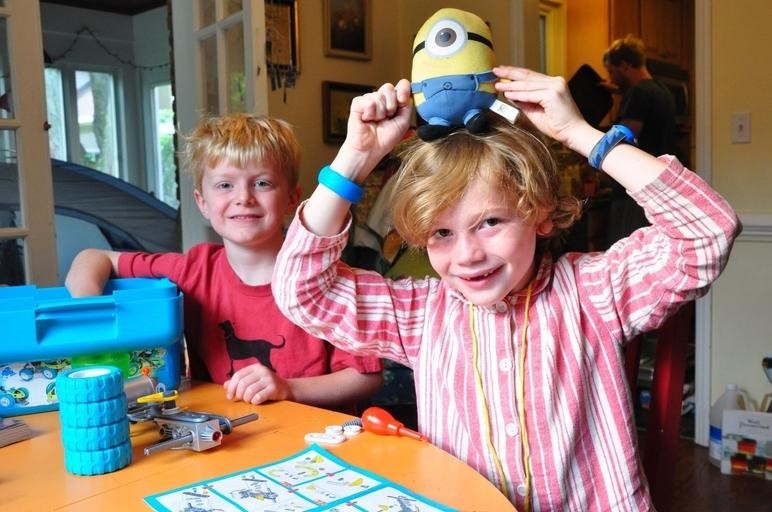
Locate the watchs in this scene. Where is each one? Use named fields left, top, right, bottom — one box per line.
left=588, top=125, right=636, bottom=171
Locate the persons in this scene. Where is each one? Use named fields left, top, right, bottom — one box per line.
left=65, top=114, right=384, bottom=404
left=272, top=67, right=740, bottom=512
left=584, top=36, right=679, bottom=251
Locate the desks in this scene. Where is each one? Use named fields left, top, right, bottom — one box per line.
left=0, top=382, right=520, bottom=512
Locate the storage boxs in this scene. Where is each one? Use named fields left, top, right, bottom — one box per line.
left=1, top=278, right=186, bottom=418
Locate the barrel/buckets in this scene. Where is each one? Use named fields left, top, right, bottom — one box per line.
left=705, top=384, right=754, bottom=469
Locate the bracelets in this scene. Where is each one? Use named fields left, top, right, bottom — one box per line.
left=316, top=166, right=364, bottom=204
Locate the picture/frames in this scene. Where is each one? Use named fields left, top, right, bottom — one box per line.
left=263, top=1, right=377, bottom=147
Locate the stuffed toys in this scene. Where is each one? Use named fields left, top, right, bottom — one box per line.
left=409, top=8, right=514, bottom=142
